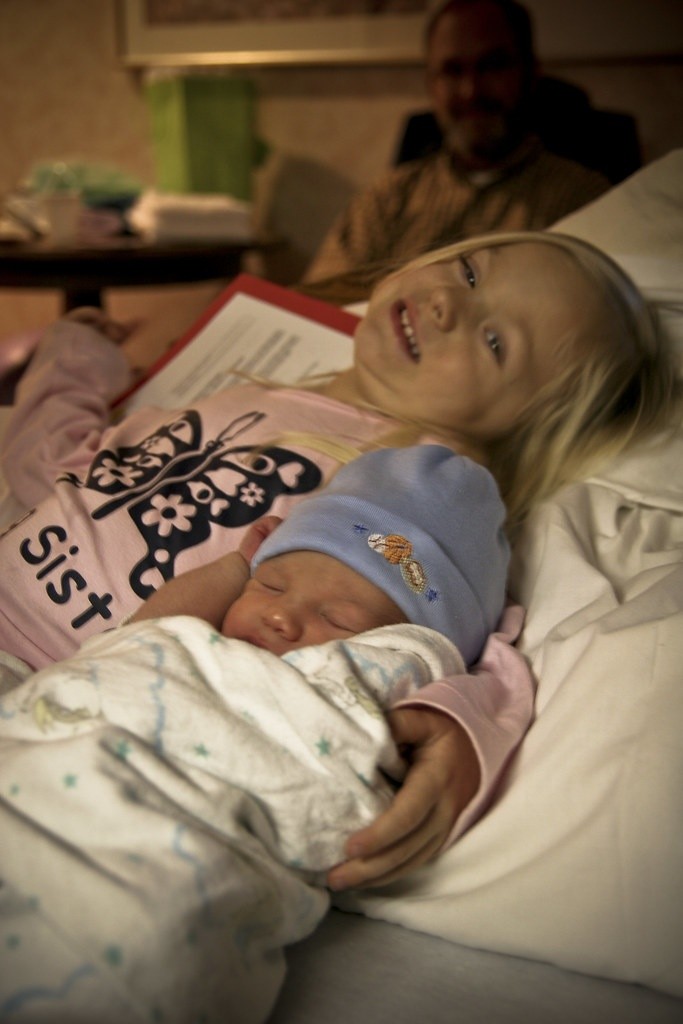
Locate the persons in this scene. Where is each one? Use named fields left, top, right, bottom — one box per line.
left=294, top=2, right=611, bottom=283
left=0, top=447, right=515, bottom=1024
left=0, top=232, right=666, bottom=898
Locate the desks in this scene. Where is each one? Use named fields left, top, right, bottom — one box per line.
left=0, top=237, right=283, bottom=406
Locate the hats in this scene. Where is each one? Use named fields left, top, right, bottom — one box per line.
left=251, top=445, right=511, bottom=670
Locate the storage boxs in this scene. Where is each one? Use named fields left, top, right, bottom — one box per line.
left=150, top=69, right=253, bottom=202
left=248, top=140, right=315, bottom=241
